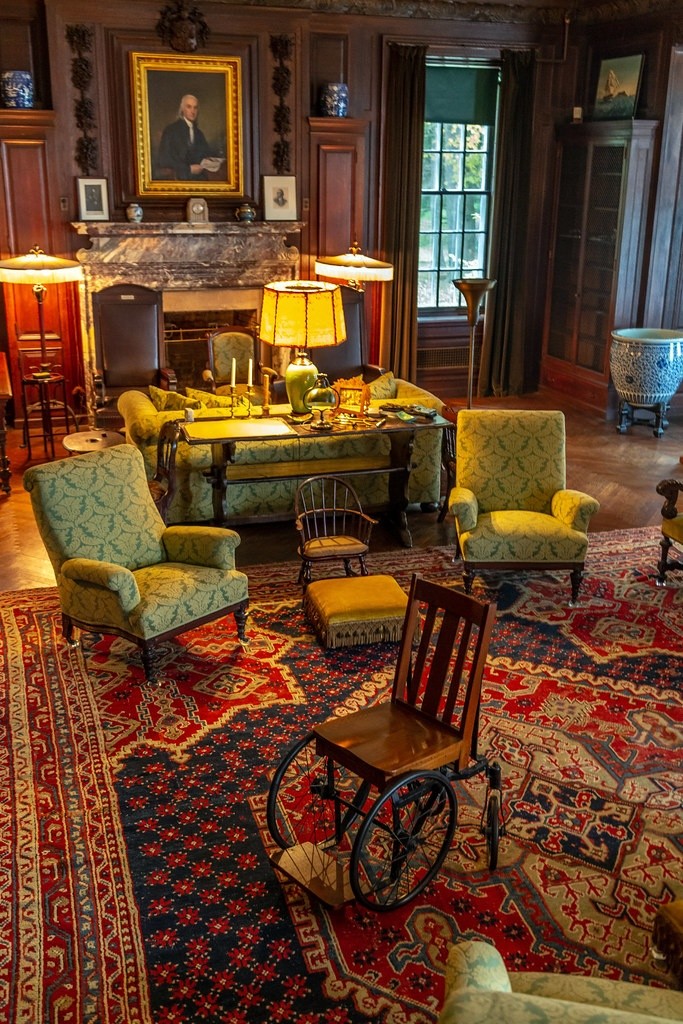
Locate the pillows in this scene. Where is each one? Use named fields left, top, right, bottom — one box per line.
left=147, top=371, right=396, bottom=411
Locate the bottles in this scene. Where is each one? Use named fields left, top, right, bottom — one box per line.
left=321, top=82, right=349, bottom=117
left=126, top=203, right=143, bottom=223
left=0, top=69, right=35, bottom=108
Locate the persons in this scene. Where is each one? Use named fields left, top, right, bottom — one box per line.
left=274, top=188, right=288, bottom=206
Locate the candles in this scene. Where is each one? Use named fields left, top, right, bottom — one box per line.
left=248, top=359, right=252, bottom=385
left=231, top=357, right=236, bottom=387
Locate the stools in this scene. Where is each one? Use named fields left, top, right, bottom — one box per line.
left=305, top=575, right=420, bottom=648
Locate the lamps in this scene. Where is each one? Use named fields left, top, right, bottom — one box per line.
left=0, top=245, right=83, bottom=472
left=258, top=242, right=394, bottom=414
left=454, top=278, right=497, bottom=413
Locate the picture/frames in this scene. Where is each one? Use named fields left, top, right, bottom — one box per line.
left=77, top=49, right=297, bottom=222
left=332, top=377, right=370, bottom=417
left=586, top=52, right=646, bottom=119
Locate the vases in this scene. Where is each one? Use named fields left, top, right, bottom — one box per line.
left=0, top=71, right=34, bottom=110
left=319, top=83, right=350, bottom=118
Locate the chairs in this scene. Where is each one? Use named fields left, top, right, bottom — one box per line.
left=656, top=480, right=683, bottom=583
left=437, top=940, right=683, bottom=1024
left=21, top=408, right=601, bottom=910
left=273, top=285, right=386, bottom=405
left=85, top=284, right=257, bottom=431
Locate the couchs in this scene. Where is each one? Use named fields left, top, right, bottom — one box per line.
left=116, top=379, right=447, bottom=524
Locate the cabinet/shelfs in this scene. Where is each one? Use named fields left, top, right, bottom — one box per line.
left=538, top=120, right=660, bottom=420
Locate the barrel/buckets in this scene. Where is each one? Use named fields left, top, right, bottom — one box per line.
left=609, top=328, right=683, bottom=407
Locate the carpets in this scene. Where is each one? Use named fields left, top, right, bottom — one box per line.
left=0, top=525, right=683, bottom=1024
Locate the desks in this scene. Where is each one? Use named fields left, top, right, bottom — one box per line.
left=150, top=410, right=455, bottom=551
left=62, top=430, right=125, bottom=457
